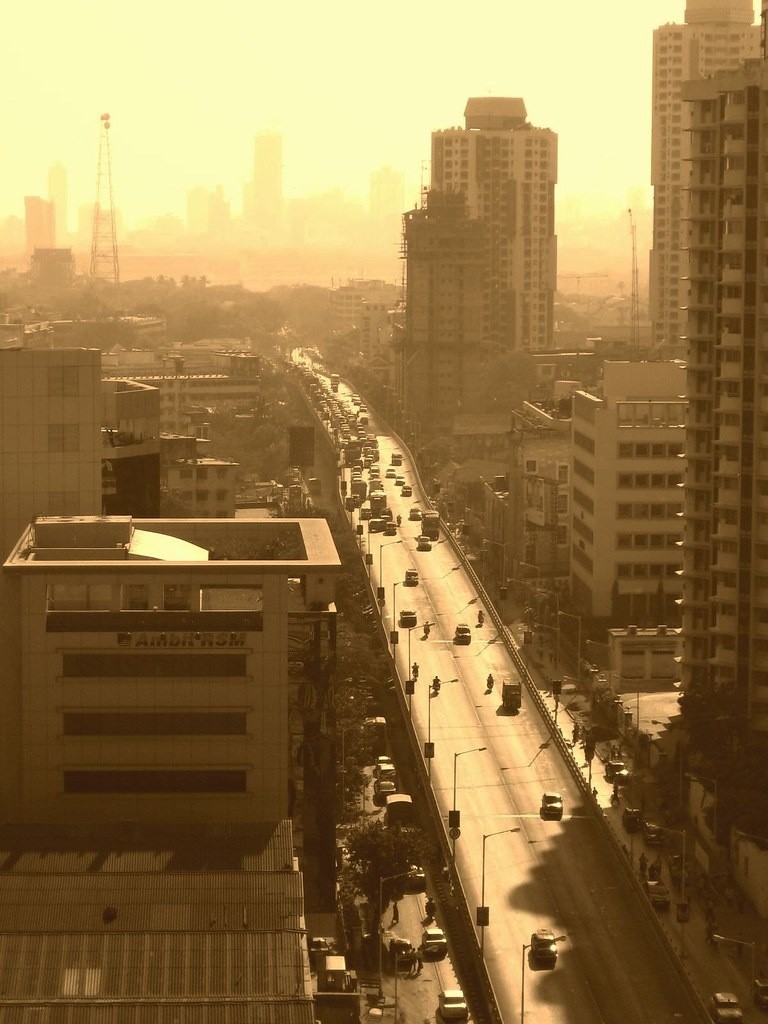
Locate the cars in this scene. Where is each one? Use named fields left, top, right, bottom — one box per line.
left=361, top=718, right=449, bottom=960
left=437, top=988, right=469, bottom=1021
left=645, top=881, right=669, bottom=907
left=753, top=977, right=768, bottom=1014
left=541, top=793, right=564, bottom=821
left=303, top=366, right=691, bottom=924
left=529, top=930, right=560, bottom=967
left=713, top=991, right=744, bottom=1024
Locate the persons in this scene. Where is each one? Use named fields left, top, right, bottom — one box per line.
left=397, top=515, right=402, bottom=523
left=478, top=610, right=482, bottom=618
left=425, top=897, right=435, bottom=914
left=412, top=661, right=418, bottom=676
left=409, top=947, right=423, bottom=974
left=538, top=633, right=562, bottom=667
left=487, top=674, right=493, bottom=686
left=424, top=621, right=430, bottom=630
left=433, top=676, right=440, bottom=688
left=392, top=901, right=400, bottom=924
left=546, top=674, right=553, bottom=697
left=571, top=720, right=749, bottom=959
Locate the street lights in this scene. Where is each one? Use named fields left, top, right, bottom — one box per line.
left=342, top=716, right=380, bottom=811
left=650, top=720, right=685, bottom=818
left=358, top=495, right=438, bottom=716
left=682, top=770, right=718, bottom=859
left=377, top=869, right=418, bottom=997
left=427, top=679, right=460, bottom=781
left=448, top=481, right=506, bottom=587
left=394, top=945, right=440, bottom=1024
left=712, top=935, right=756, bottom=1014
left=480, top=827, right=521, bottom=956
left=556, top=611, right=582, bottom=679
left=452, top=746, right=487, bottom=862
left=361, top=770, right=398, bottom=831
left=612, top=672, right=640, bottom=760
left=517, top=560, right=541, bottom=635
left=519, top=932, right=568, bottom=1023
left=648, top=823, right=688, bottom=904
